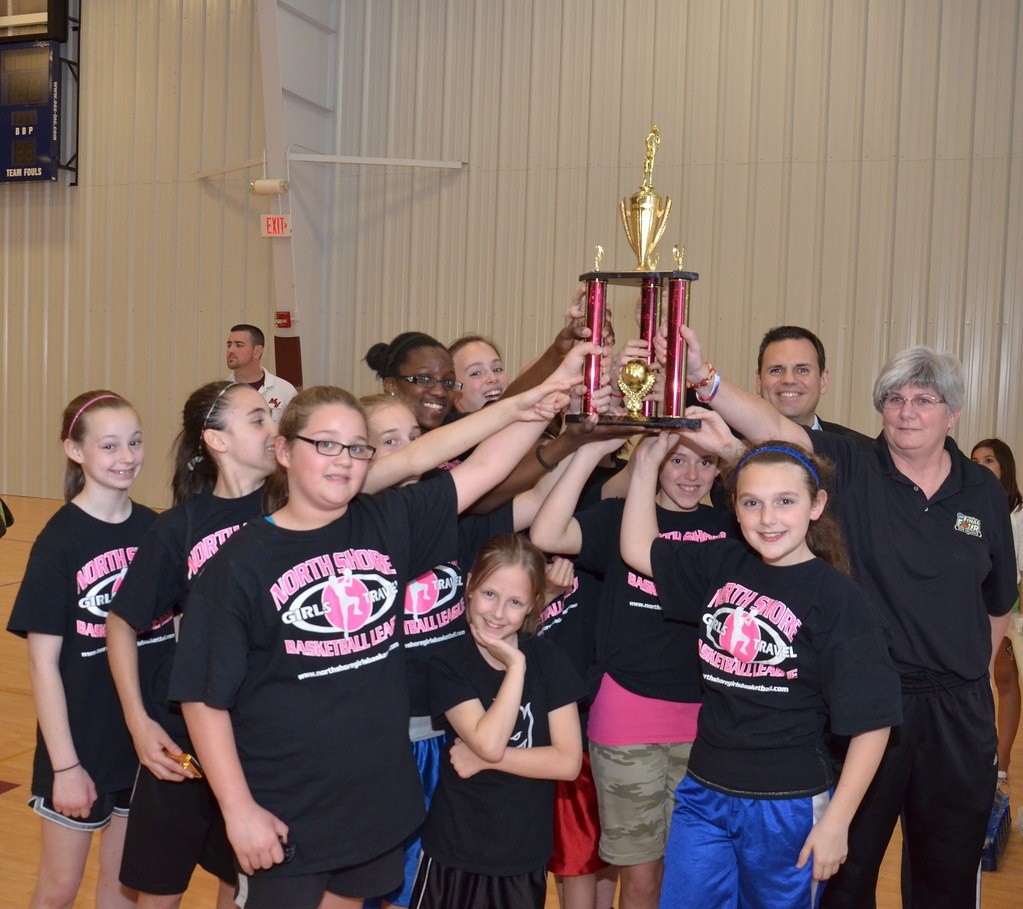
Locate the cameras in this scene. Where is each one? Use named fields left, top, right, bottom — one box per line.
left=165, top=751, right=205, bottom=779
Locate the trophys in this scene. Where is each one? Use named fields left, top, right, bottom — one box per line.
left=566, top=125, right=701, bottom=428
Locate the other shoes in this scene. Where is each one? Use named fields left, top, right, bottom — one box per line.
left=998, top=771, right=1012, bottom=795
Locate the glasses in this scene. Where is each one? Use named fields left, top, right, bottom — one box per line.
left=391, top=374, right=464, bottom=392
left=294, top=434, right=376, bottom=460
left=879, top=394, right=946, bottom=410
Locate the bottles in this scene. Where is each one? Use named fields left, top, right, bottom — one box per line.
left=983, top=771, right=1010, bottom=854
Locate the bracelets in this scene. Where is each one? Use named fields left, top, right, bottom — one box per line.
left=54, top=761, right=81, bottom=773
left=696, top=372, right=720, bottom=403
left=686, top=362, right=715, bottom=388
left=536, top=445, right=558, bottom=469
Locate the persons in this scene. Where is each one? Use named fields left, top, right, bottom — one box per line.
left=106, top=285, right=856, bottom=908
left=654, top=323, right=1018, bottom=908
left=225, top=324, right=298, bottom=421
left=970, top=439, right=1023, bottom=797
left=6, top=390, right=160, bottom=909
left=620, top=430, right=903, bottom=909
